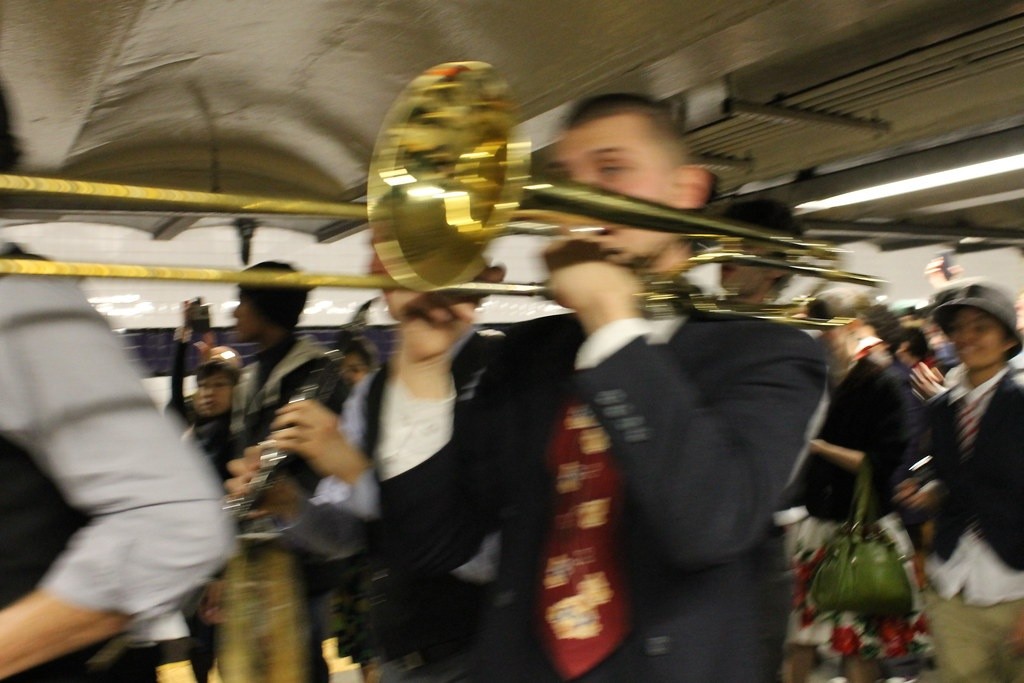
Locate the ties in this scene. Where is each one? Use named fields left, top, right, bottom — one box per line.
left=539, top=395, right=630, bottom=680
left=954, top=380, right=1001, bottom=540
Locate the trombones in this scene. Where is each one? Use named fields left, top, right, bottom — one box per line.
left=0, top=56, right=890, bottom=332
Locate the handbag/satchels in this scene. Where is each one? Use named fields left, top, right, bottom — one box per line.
left=803, top=456, right=909, bottom=615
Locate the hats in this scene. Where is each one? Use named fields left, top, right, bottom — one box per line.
left=930, top=283, right=1023, bottom=362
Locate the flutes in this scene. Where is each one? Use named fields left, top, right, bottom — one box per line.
left=223, top=345, right=351, bottom=526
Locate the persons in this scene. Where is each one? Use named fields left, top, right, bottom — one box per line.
left=165, top=91, right=1024, bottom=682
left=909, top=281, right=1024, bottom=683
left=0, top=85, right=234, bottom=683
